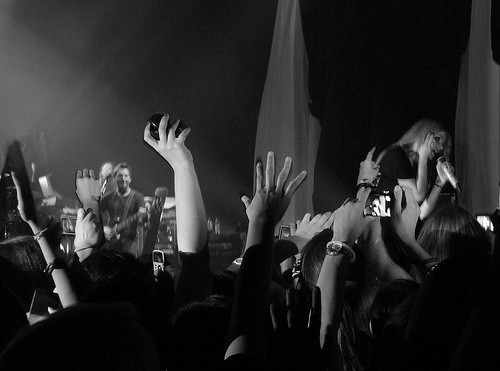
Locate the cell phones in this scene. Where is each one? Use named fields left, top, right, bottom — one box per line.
left=153, top=249, right=165, bottom=281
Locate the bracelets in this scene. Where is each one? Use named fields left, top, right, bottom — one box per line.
left=425, top=263, right=439, bottom=275
left=33, top=227, right=48, bottom=241
left=326, top=240, right=356, bottom=260
left=43, top=256, right=64, bottom=275
left=434, top=181, right=442, bottom=189
left=356, top=178, right=374, bottom=192
left=424, top=257, right=438, bottom=265
left=73, top=245, right=98, bottom=251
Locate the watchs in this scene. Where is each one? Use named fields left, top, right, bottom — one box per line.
left=324, top=242, right=354, bottom=259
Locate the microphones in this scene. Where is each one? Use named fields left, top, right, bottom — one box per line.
left=437, top=156, right=460, bottom=189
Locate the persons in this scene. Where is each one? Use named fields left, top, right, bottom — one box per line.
left=0, top=113, right=500, bottom=371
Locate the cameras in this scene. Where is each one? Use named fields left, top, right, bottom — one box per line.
left=148, top=112, right=186, bottom=141
left=361, top=188, right=396, bottom=217
left=279, top=225, right=291, bottom=240
left=60, top=215, right=77, bottom=236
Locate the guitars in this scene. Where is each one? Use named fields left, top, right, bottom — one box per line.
left=103, top=207, right=148, bottom=247
